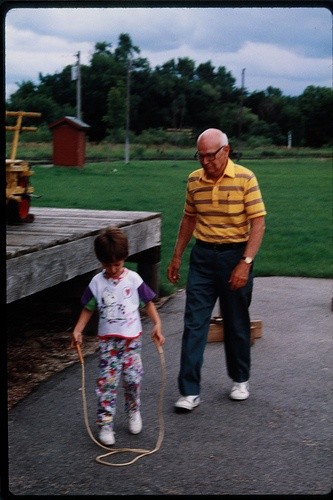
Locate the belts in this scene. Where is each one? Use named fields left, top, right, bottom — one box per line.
left=195, top=241, right=247, bottom=251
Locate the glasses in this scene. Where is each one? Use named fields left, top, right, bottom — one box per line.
left=194, top=145, right=225, bottom=162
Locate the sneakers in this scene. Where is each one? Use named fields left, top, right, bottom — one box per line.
left=230, top=379, right=250, bottom=399
left=129, top=411, right=142, bottom=434
left=174, top=395, right=200, bottom=410
left=100, top=424, right=115, bottom=444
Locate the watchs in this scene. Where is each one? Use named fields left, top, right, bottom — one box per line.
left=240, top=256, right=254, bottom=266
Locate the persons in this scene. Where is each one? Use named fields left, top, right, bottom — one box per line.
left=165, top=128, right=268, bottom=413
left=69, top=227, right=165, bottom=445
